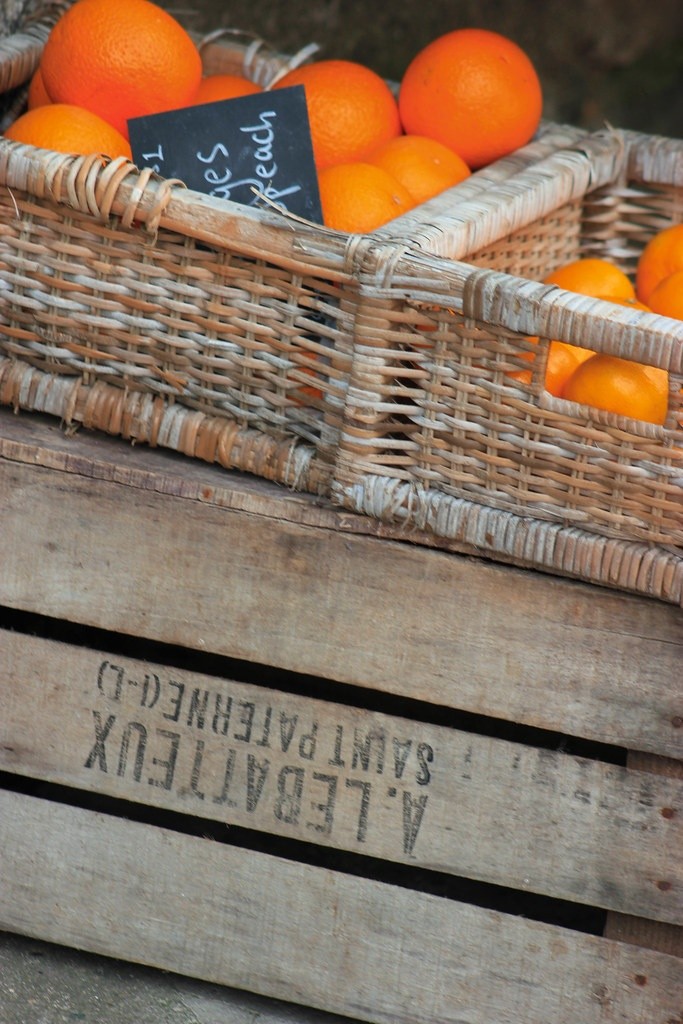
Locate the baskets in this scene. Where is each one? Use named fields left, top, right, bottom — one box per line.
left=333, top=125, right=683, bottom=608
left=0, top=8, right=588, bottom=498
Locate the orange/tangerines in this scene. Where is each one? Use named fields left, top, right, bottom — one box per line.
left=505, top=224, right=683, bottom=432
left=4, top=0, right=541, bottom=235
